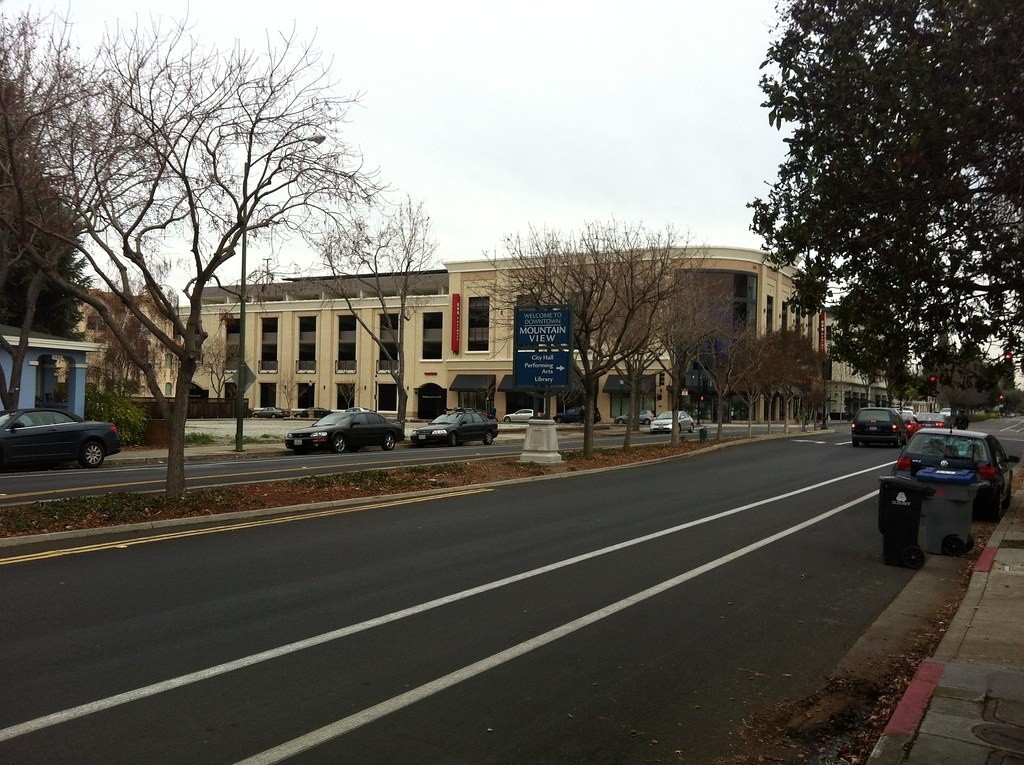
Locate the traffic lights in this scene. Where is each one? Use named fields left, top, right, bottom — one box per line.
left=660, top=371, right=665, bottom=386
left=930, top=375, right=937, bottom=392
left=703, top=379, right=709, bottom=391
left=1005, top=353, right=1012, bottom=360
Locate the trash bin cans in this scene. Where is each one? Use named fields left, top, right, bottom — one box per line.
left=878, top=474, right=935, bottom=569
left=699, top=427, right=708, bottom=444
left=919, top=467, right=977, bottom=555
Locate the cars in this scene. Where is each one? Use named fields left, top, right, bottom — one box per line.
left=891, top=427, right=1020, bottom=522
left=294, top=407, right=331, bottom=418
left=1, top=408, right=121, bottom=467
left=410, top=407, right=499, bottom=447
left=851, top=408, right=908, bottom=448
left=251, top=407, right=290, bottom=418
left=345, top=407, right=376, bottom=412
left=553, top=407, right=601, bottom=424
left=941, top=408, right=952, bottom=417
left=503, top=409, right=545, bottom=422
left=917, top=413, right=952, bottom=429
left=900, top=413, right=921, bottom=436
left=284, top=412, right=405, bottom=454
left=651, top=410, right=694, bottom=434
left=614, top=409, right=654, bottom=425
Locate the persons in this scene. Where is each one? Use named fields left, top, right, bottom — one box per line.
left=795, top=413, right=801, bottom=425
left=492, top=405, right=498, bottom=422
left=804, top=413, right=809, bottom=424
left=954, top=409, right=970, bottom=430
left=579, top=406, right=585, bottom=424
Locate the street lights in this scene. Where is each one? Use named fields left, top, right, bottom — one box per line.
left=234, top=136, right=326, bottom=452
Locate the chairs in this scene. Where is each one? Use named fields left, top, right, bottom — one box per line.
left=922, top=438, right=985, bottom=459
